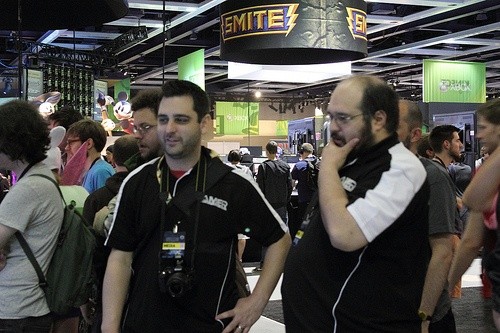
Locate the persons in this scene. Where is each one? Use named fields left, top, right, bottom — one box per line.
left=0, top=75, right=500, bottom=333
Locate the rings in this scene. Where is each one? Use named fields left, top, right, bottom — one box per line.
left=237, top=325, right=244, bottom=330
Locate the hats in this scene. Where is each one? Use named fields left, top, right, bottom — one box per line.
left=106, top=145, right=114, bottom=154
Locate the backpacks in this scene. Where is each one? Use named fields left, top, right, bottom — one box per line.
left=14, top=174, right=107, bottom=317
left=295, top=157, right=318, bottom=193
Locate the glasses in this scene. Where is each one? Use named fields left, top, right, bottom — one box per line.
left=65, top=139, right=80, bottom=148
left=132, top=123, right=156, bottom=134
left=325, top=111, right=374, bottom=124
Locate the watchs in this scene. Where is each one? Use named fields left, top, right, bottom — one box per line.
left=418, top=310, right=432, bottom=321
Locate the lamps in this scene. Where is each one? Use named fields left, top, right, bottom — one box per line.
left=384, top=73, right=421, bottom=98
left=3, top=77, right=13, bottom=94
left=268, top=89, right=332, bottom=114
